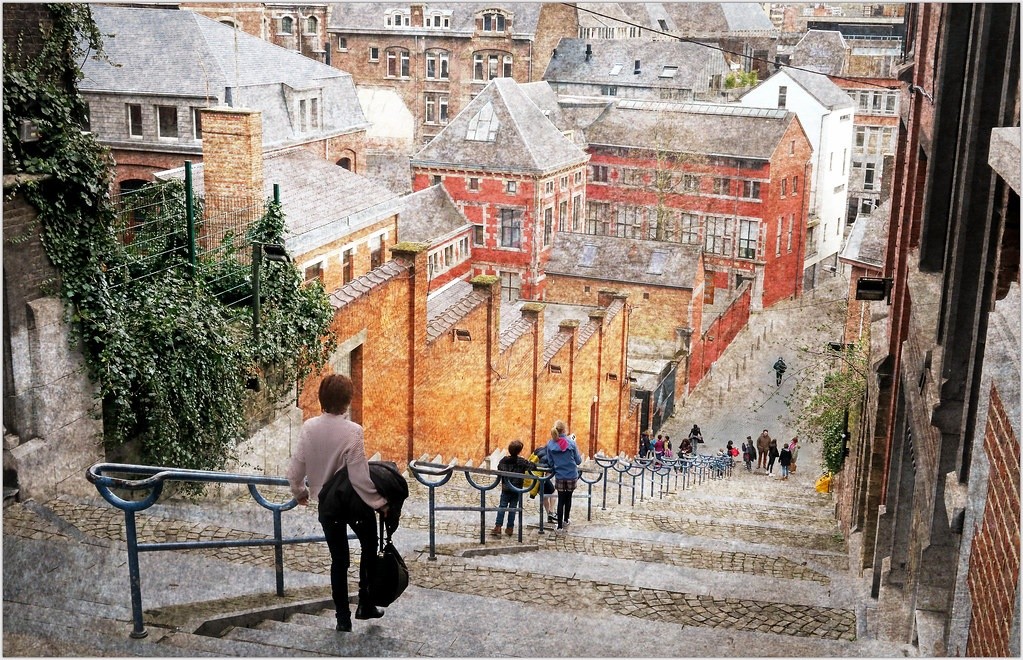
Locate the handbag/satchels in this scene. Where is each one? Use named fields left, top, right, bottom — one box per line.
left=744, top=452, right=751, bottom=462
left=789, top=463, right=796, bottom=472
left=369, top=512, right=409, bottom=608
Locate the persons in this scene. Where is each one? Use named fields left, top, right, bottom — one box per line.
left=778, top=443, right=792, bottom=479
left=717, top=449, right=725, bottom=477
left=727, top=441, right=736, bottom=466
left=688, top=424, right=704, bottom=444
left=547, top=421, right=582, bottom=536
left=640, top=432, right=672, bottom=467
left=676, top=439, right=693, bottom=473
left=742, top=437, right=755, bottom=470
left=491, top=440, right=537, bottom=536
left=524, top=442, right=558, bottom=524
left=757, top=430, right=771, bottom=469
left=288, top=374, right=385, bottom=632
left=789, top=437, right=801, bottom=474
left=773, top=357, right=787, bottom=387
left=767, top=439, right=779, bottom=476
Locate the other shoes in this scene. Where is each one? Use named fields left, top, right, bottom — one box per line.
left=505, top=528, right=513, bottom=534
left=554, top=527, right=564, bottom=535
left=766, top=470, right=769, bottom=474
left=491, top=525, right=501, bottom=533
left=335, top=624, right=351, bottom=632
left=563, top=520, right=571, bottom=527
left=548, top=516, right=552, bottom=522
left=355, top=608, right=384, bottom=619
left=769, top=473, right=775, bottom=477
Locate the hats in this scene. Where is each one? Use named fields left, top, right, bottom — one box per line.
left=793, top=437, right=798, bottom=443
